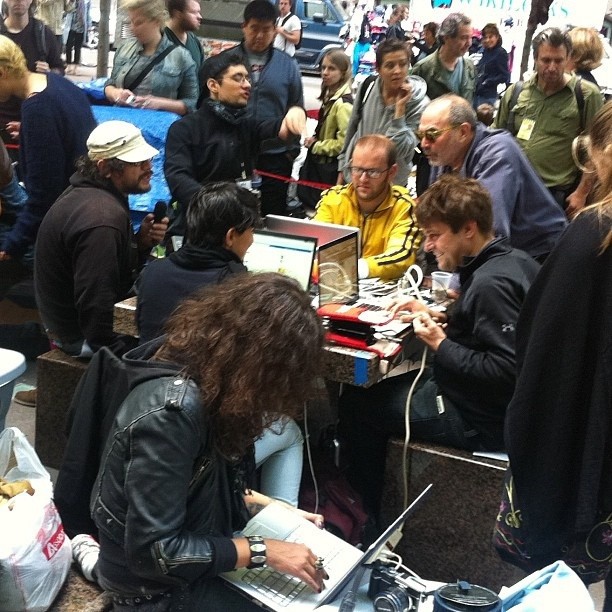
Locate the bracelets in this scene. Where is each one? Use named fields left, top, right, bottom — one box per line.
left=242, top=535, right=268, bottom=570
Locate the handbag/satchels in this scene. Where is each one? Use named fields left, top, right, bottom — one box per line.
left=493, top=206, right=612, bottom=583
left=296, top=94, right=355, bottom=207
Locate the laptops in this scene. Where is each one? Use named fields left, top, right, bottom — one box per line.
left=242, top=229, right=319, bottom=292
left=214, top=482, right=434, bottom=612
left=316, top=231, right=413, bottom=339
left=265, top=213, right=361, bottom=260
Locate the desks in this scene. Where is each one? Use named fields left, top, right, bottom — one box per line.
left=113, top=293, right=460, bottom=402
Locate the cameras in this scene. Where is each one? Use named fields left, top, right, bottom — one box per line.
left=367, top=562, right=427, bottom=612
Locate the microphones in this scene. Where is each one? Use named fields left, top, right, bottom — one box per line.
left=152, top=200, right=168, bottom=224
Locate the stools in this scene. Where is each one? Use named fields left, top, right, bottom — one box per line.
left=34, top=348, right=85, bottom=471
left=378, top=436, right=515, bottom=541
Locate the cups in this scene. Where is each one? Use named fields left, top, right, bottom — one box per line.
left=430, top=270, right=451, bottom=303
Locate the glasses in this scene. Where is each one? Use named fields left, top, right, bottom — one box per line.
left=349, top=165, right=389, bottom=179
left=215, top=75, right=252, bottom=83
left=414, top=125, right=462, bottom=142
left=126, top=158, right=153, bottom=167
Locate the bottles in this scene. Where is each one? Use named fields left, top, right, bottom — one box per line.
left=71, top=534, right=101, bottom=582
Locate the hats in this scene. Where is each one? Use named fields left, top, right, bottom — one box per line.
left=87, top=120, right=160, bottom=162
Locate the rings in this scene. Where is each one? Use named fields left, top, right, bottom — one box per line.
left=314, top=555, right=324, bottom=574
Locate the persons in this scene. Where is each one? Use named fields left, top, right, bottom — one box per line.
left=63, top=0, right=92, bottom=66
left=0, top=34, right=100, bottom=214
left=205, top=0, right=305, bottom=217
left=416, top=24, right=440, bottom=54
left=273, top=0, right=301, bottom=59
left=494, top=29, right=603, bottom=221
left=89, top=272, right=329, bottom=612
left=134, top=182, right=258, bottom=346
left=475, top=22, right=512, bottom=98
left=34, top=0, right=65, bottom=35
left=104, top=0, right=199, bottom=115
left=13, top=120, right=170, bottom=406
left=410, top=13, right=475, bottom=101
left=392, top=177, right=546, bottom=454
left=0, top=136, right=29, bottom=265
left=336, top=39, right=432, bottom=189
left=386, top=2, right=407, bottom=43
left=406, top=20, right=424, bottom=42
left=492, top=100, right=612, bottom=612
left=411, top=94, right=570, bottom=266
left=160, top=0, right=205, bottom=73
left=345, top=1, right=373, bottom=78
left=311, top=134, right=423, bottom=280
left=163, top=53, right=262, bottom=237
left=297, top=49, right=357, bottom=209
left=0, top=0, right=68, bottom=78
left=563, top=28, right=606, bottom=91
